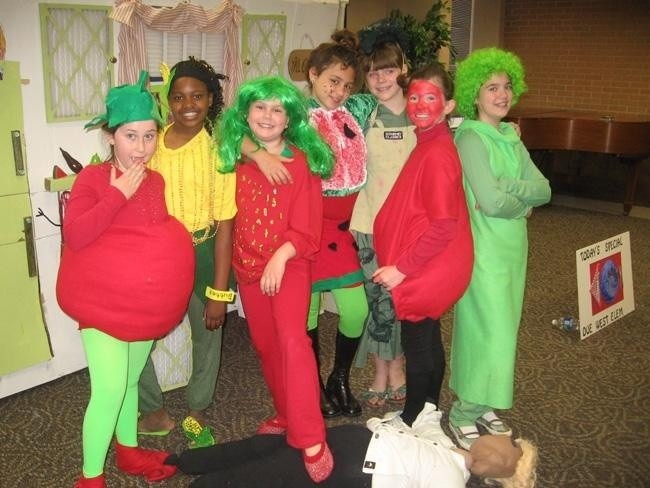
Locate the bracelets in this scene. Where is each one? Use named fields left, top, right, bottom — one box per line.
left=204, top=287, right=236, bottom=304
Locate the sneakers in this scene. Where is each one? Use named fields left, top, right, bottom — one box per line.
left=113, top=439, right=177, bottom=481
left=77, top=472, right=105, bottom=488
left=448, top=421, right=480, bottom=450
left=368, top=412, right=412, bottom=435
left=477, top=412, right=512, bottom=436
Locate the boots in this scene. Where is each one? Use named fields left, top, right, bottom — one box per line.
left=326, top=328, right=362, bottom=416
left=306, top=326, right=341, bottom=417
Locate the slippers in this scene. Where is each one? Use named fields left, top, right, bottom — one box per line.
left=364, top=383, right=388, bottom=408
left=386, top=384, right=407, bottom=404
left=138, top=411, right=170, bottom=436
left=181, top=416, right=215, bottom=449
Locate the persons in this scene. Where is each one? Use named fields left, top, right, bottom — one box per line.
left=138, top=56, right=238, bottom=449
left=447, top=44, right=554, bottom=451
left=54, top=68, right=197, bottom=488
left=349, top=38, right=417, bottom=419
left=366, top=61, right=474, bottom=437
left=163, top=403, right=542, bottom=488
left=240, top=28, right=370, bottom=418
left=211, top=73, right=338, bottom=484
